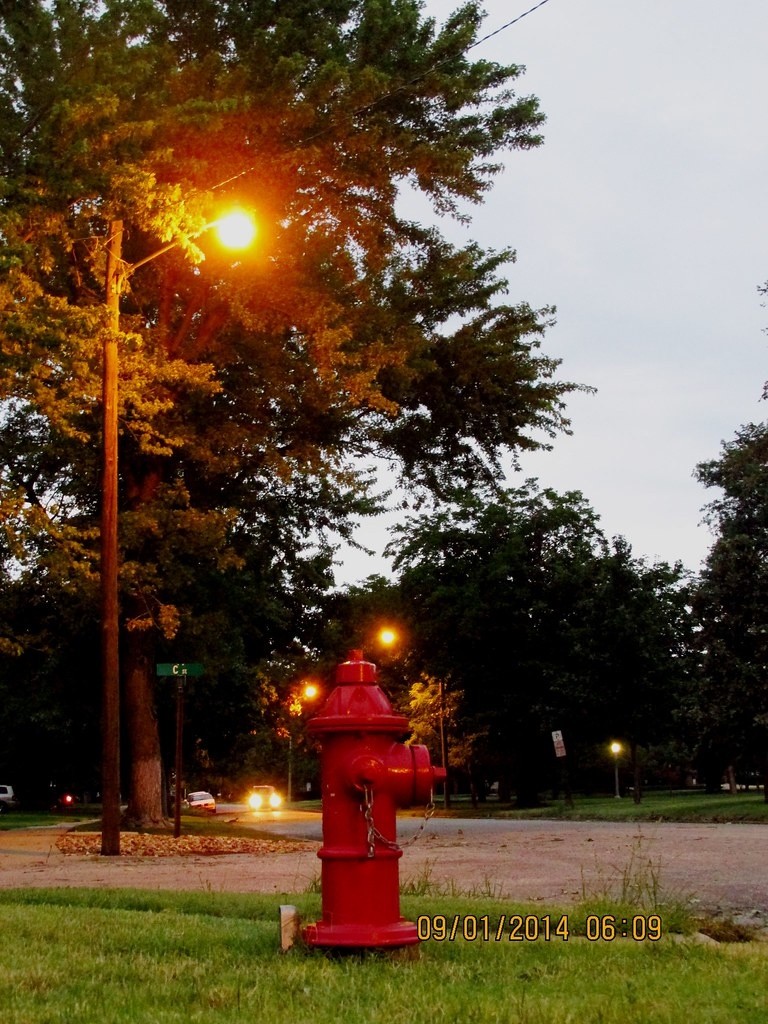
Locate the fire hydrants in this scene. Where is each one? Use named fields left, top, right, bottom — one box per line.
left=288, top=649, right=452, bottom=951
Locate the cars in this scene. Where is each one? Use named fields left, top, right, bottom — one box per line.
left=183, top=790, right=217, bottom=815
left=0, top=784, right=18, bottom=815
left=247, top=784, right=280, bottom=812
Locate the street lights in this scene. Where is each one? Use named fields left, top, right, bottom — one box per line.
left=610, top=739, right=625, bottom=799
